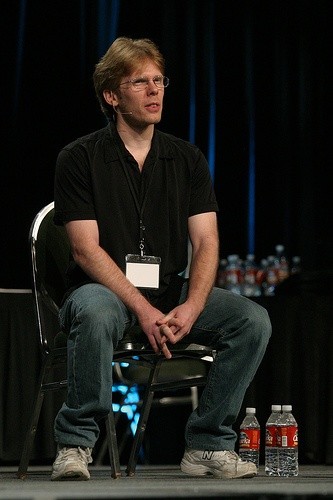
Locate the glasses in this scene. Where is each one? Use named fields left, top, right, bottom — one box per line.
left=116, top=75, right=170, bottom=90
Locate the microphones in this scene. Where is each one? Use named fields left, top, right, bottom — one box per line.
left=113, top=106, right=132, bottom=115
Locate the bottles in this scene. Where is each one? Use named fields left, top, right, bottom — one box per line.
left=276, top=405, right=298, bottom=477
left=265, top=405, right=283, bottom=477
left=218, top=246, right=299, bottom=297
left=239, top=408, right=259, bottom=474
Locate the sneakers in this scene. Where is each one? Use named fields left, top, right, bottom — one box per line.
left=50, top=446, right=94, bottom=482
left=179, top=448, right=258, bottom=480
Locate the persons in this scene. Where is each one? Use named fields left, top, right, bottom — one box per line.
left=52, top=38, right=273, bottom=480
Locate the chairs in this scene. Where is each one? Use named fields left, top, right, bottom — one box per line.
left=113, top=357, right=212, bottom=412
left=17, top=201, right=214, bottom=479
